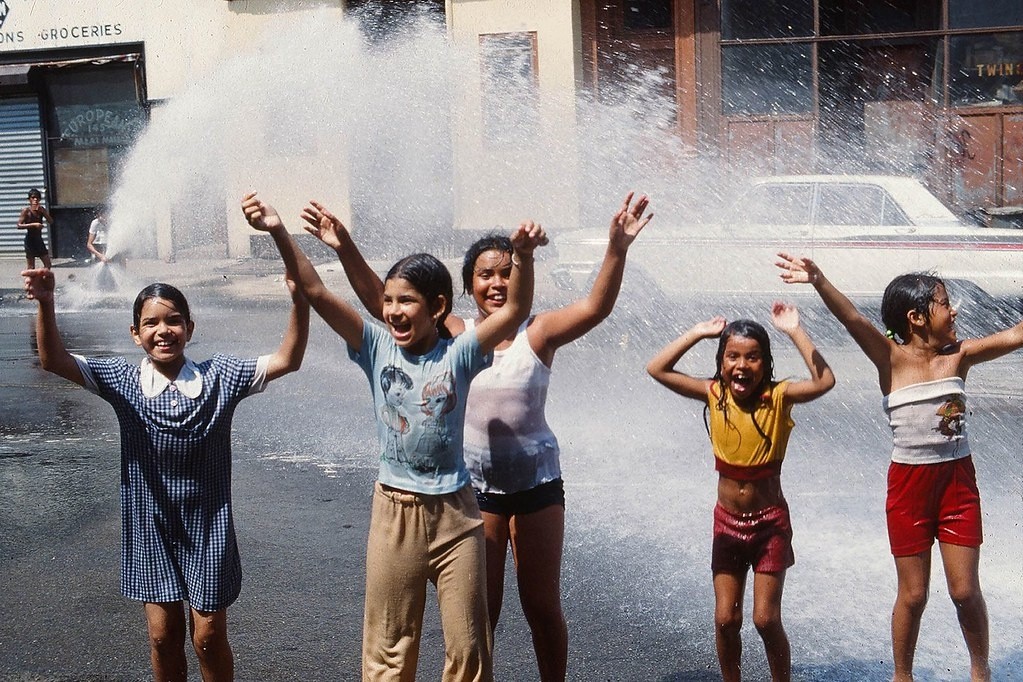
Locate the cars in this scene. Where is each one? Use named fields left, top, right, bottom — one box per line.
left=557, top=169, right=1022, bottom=342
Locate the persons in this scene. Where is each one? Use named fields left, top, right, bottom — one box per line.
left=647, top=300, right=837, bottom=682
left=240, top=188, right=550, bottom=682
left=17, top=189, right=52, bottom=271
left=302, top=190, right=653, bottom=682
left=21, top=266, right=311, bottom=682
left=87, top=204, right=110, bottom=261
left=774, top=252, right=1023, bottom=682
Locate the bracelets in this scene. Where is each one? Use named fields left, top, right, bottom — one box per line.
left=511, top=254, right=536, bottom=266
